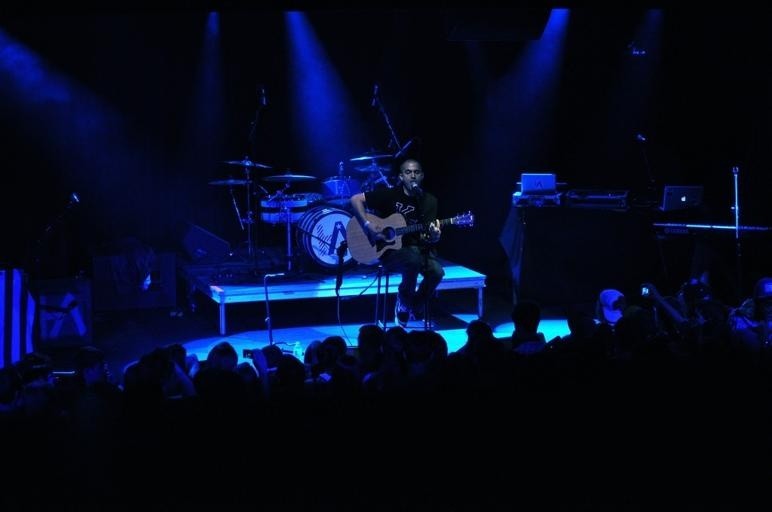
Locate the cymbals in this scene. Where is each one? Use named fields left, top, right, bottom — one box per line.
left=349, top=154, right=392, bottom=161
left=265, top=175, right=316, bottom=180
left=223, top=161, right=272, bottom=169
left=211, top=181, right=252, bottom=184
left=352, top=166, right=391, bottom=172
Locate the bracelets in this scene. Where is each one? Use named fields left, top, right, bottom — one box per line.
left=362, top=220, right=370, bottom=227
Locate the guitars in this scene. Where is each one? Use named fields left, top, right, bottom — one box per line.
left=346, top=210, right=474, bottom=264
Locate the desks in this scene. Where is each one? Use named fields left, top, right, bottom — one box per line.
left=189, top=263, right=487, bottom=336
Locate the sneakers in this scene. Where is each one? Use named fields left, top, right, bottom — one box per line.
left=413, top=306, right=425, bottom=320
left=395, top=292, right=410, bottom=325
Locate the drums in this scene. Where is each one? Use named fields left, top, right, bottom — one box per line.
left=321, top=175, right=362, bottom=205
left=297, top=205, right=354, bottom=267
left=261, top=194, right=307, bottom=222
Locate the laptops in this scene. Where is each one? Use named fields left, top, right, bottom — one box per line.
left=658, top=184, right=702, bottom=211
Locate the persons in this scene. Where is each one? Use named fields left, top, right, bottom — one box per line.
left=351, top=159, right=444, bottom=330
left=1, top=272, right=772, bottom=511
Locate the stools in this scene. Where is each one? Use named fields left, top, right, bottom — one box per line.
left=374, top=264, right=434, bottom=332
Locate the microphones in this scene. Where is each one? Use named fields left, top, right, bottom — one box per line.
left=409, top=177, right=423, bottom=197
left=259, top=86, right=268, bottom=106
left=371, top=80, right=382, bottom=108
